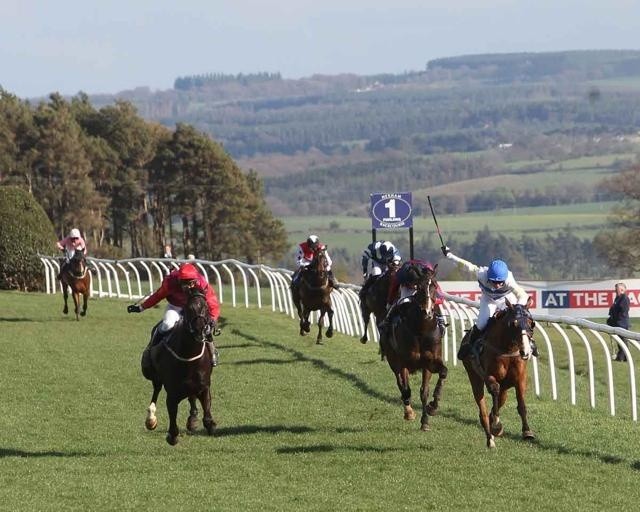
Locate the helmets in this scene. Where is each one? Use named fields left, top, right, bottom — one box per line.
left=177, top=263, right=198, bottom=279
left=373, top=241, right=394, bottom=263
left=487, top=259, right=507, bottom=282
left=308, top=235, right=319, bottom=246
left=70, top=229, right=80, bottom=239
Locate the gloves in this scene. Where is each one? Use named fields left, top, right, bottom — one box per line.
left=127, top=305, right=141, bottom=313
left=441, top=245, right=450, bottom=256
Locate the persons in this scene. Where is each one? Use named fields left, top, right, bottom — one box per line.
left=55, top=228, right=87, bottom=280
left=606, top=282, right=630, bottom=362
left=291, top=234, right=340, bottom=290
left=127, top=263, right=220, bottom=367
left=442, top=245, right=538, bottom=362
left=384, top=259, right=451, bottom=328
left=160, top=244, right=177, bottom=279
left=361, top=239, right=401, bottom=280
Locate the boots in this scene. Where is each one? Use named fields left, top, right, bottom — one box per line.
left=86, top=261, right=97, bottom=275
left=206, top=334, right=218, bottom=365
left=145, top=327, right=164, bottom=354
left=57, top=261, right=70, bottom=280
left=458, top=325, right=480, bottom=361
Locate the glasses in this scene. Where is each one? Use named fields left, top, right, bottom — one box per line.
left=181, top=281, right=193, bottom=286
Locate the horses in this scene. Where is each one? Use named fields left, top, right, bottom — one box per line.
left=145, top=283, right=217, bottom=445
left=358, top=260, right=402, bottom=344
left=290, top=245, right=334, bottom=345
left=379, top=263, right=449, bottom=432
left=59, top=247, right=90, bottom=321
left=457, top=296, right=535, bottom=449
